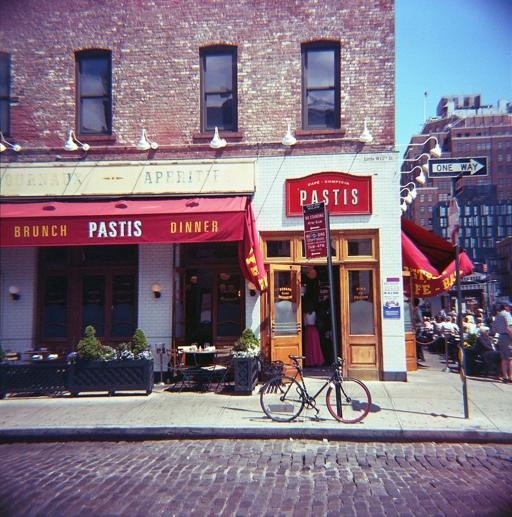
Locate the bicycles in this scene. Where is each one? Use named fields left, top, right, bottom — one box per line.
left=256, top=354, right=373, bottom=424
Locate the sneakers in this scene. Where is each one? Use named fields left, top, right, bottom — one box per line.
left=418, top=358, right=425, bottom=362
left=503, top=377, right=512, bottom=383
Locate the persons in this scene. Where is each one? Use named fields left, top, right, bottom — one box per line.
left=303, top=299, right=326, bottom=367
left=412, top=298, right=426, bottom=362
left=423, top=299, right=511, bottom=384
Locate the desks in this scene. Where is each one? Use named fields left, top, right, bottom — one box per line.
left=24, top=350, right=52, bottom=359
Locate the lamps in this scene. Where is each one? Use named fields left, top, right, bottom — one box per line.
left=210, top=127, right=227, bottom=149
left=0, top=131, right=21, bottom=153
left=248, top=281, right=257, bottom=296
left=136, top=128, right=159, bottom=151
left=64, top=130, right=90, bottom=151
left=152, top=283, right=160, bottom=298
left=399, top=181, right=418, bottom=212
left=281, top=122, right=297, bottom=145
left=9, top=286, right=19, bottom=300
left=398, top=153, right=431, bottom=172
left=400, top=166, right=426, bottom=184
left=397, top=135, right=441, bottom=158
left=358, top=120, right=373, bottom=143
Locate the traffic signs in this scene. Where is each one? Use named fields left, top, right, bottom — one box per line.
left=427, top=156, right=488, bottom=178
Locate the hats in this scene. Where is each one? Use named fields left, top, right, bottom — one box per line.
left=501, top=300, right=512, bottom=307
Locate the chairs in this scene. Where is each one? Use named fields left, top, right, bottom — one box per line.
left=164, top=343, right=236, bottom=393
left=427, top=323, right=500, bottom=376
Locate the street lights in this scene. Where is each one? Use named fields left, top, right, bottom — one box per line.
left=464, top=279, right=500, bottom=314
